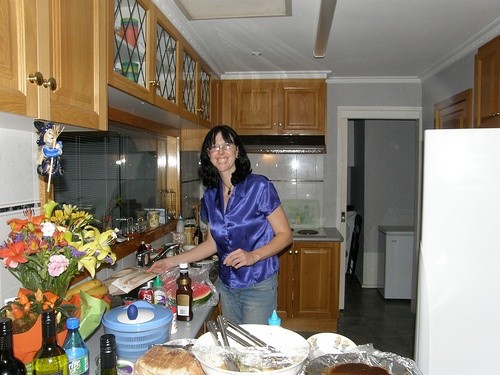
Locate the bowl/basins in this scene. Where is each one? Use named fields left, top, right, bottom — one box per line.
left=101, top=304, right=172, bottom=361
left=192, top=324, right=310, bottom=374
left=307, top=332, right=356, bottom=362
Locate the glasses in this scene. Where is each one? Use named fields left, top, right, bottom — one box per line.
left=209, top=142, right=235, bottom=152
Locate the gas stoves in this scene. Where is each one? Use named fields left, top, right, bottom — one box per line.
left=290, top=227, right=327, bottom=238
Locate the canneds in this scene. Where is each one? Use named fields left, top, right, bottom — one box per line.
left=148, top=211, right=160, bottom=228
left=138, top=286, right=154, bottom=304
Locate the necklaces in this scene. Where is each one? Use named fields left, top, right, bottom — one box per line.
left=222, top=181, right=233, bottom=194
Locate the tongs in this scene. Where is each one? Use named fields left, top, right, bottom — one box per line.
left=206, top=315, right=292, bottom=371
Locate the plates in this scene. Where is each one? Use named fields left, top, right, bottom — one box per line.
left=303, top=352, right=417, bottom=375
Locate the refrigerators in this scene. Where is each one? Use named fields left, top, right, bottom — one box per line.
left=377, top=225, right=412, bottom=300
left=413, top=126, right=500, bottom=375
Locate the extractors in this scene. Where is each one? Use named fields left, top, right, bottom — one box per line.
left=237, top=134, right=328, bottom=154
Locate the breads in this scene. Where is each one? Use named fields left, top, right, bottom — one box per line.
left=134, top=345, right=205, bottom=375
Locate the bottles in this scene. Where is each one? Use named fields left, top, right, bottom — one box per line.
left=268, top=309, right=282, bottom=326
left=194, top=225, right=203, bottom=246
left=0, top=317, right=27, bottom=375
left=62, top=317, right=90, bottom=375
left=176, top=216, right=185, bottom=235
left=175, top=263, right=193, bottom=322
left=168, top=306, right=178, bottom=334
left=99, top=334, right=118, bottom=375
left=138, top=241, right=150, bottom=267
left=153, top=275, right=167, bottom=305
left=32, top=309, right=69, bottom=375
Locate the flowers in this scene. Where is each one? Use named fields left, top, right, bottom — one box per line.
left=0, top=198, right=118, bottom=333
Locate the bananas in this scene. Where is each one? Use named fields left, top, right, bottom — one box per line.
left=65, top=279, right=108, bottom=300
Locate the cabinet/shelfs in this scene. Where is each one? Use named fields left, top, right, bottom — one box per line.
left=0, top=0, right=329, bottom=138
left=277, top=242, right=343, bottom=333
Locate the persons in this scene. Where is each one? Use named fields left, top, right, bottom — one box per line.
left=146, top=124, right=293, bottom=325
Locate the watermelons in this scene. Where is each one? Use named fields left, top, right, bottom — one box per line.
left=162, top=276, right=213, bottom=306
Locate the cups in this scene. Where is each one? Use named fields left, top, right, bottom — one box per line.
left=148, top=210, right=160, bottom=228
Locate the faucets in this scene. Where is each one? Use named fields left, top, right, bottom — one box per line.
left=146, top=243, right=179, bottom=269
